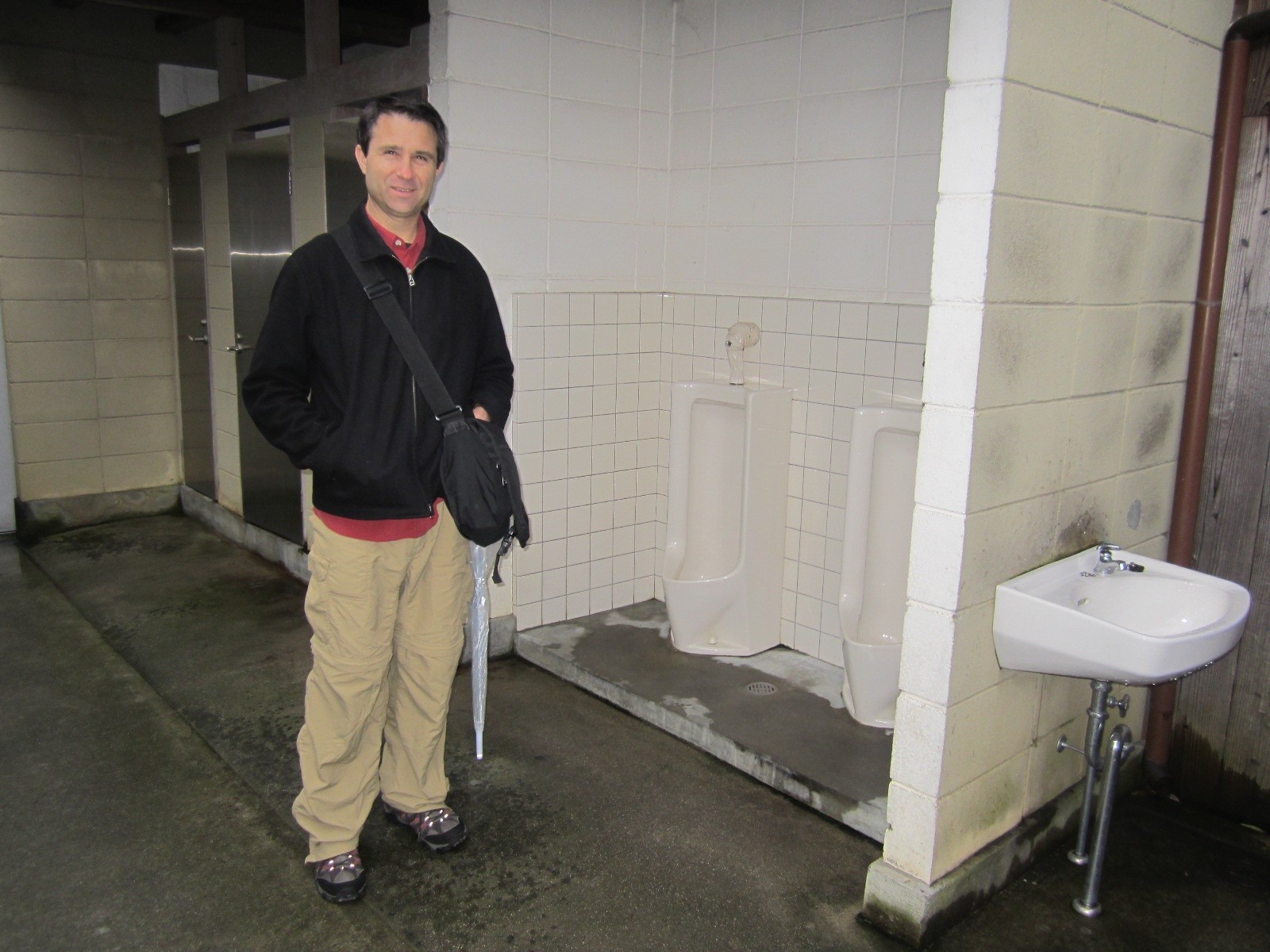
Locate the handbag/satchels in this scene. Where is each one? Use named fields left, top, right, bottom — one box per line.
left=441, top=416, right=529, bottom=583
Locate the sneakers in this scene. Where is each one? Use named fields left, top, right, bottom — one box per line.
left=312, top=847, right=368, bottom=904
left=385, top=799, right=469, bottom=850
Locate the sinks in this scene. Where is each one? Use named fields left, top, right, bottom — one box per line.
left=992, top=543, right=1250, bottom=687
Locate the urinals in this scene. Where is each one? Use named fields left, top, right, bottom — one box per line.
left=660, top=379, right=792, bottom=655
left=836, top=398, right=953, bottom=728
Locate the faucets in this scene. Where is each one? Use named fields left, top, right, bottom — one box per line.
left=1094, top=540, right=1128, bottom=575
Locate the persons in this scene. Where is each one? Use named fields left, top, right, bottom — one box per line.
left=240, top=91, right=518, bottom=909
